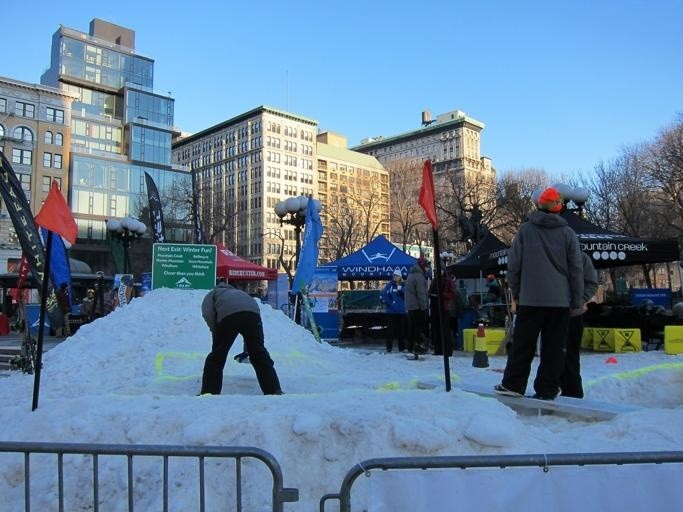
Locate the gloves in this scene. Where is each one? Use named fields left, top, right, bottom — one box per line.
left=234, top=351, right=248, bottom=363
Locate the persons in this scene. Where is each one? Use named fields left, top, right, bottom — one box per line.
left=379, top=255, right=504, bottom=360
left=79, top=288, right=100, bottom=321
left=490, top=184, right=584, bottom=401
left=57, top=281, right=72, bottom=337
left=541, top=246, right=597, bottom=397
left=200, top=282, right=282, bottom=395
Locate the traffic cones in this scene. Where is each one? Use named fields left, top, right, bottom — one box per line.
left=471, top=322, right=490, bottom=368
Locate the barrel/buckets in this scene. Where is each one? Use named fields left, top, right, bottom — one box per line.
left=307, top=308, right=340, bottom=346
left=370, top=324, right=387, bottom=341
left=345, top=325, right=364, bottom=345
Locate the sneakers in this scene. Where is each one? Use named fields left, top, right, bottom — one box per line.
left=493, top=384, right=523, bottom=398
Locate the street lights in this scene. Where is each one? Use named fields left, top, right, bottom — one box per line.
left=439, top=252, right=453, bottom=268
left=534, top=183, right=586, bottom=220
left=273, top=195, right=322, bottom=325
left=106, top=217, right=147, bottom=273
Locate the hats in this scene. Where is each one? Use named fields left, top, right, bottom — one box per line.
left=393, top=270, right=401, bottom=276
left=417, top=257, right=425, bottom=267
left=538, top=188, right=562, bottom=212
left=440, top=260, right=445, bottom=269
